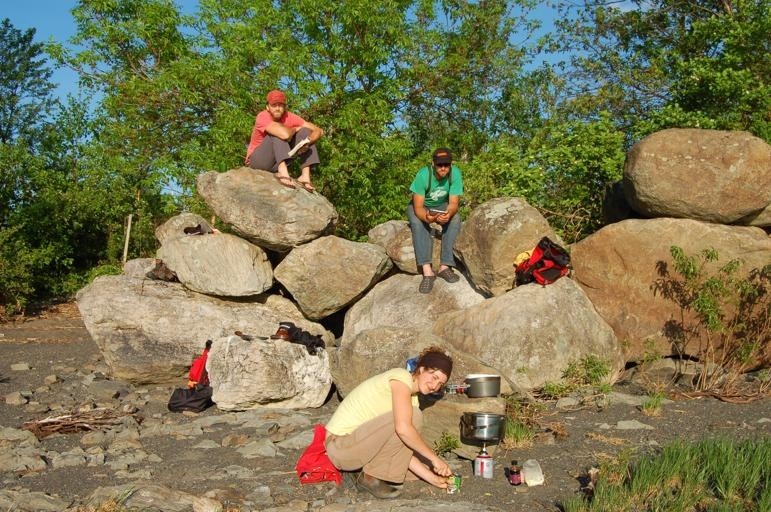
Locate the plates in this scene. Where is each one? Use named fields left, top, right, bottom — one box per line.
left=271, top=335, right=290, bottom=339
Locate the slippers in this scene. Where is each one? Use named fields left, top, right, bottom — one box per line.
left=274, top=172, right=296, bottom=189
left=295, top=178, right=315, bottom=194
left=419, top=276, right=437, bottom=293
left=438, top=268, right=460, bottom=283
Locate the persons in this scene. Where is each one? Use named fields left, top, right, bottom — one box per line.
left=324, top=345, right=464, bottom=499
left=405, top=147, right=464, bottom=295
left=245, top=89, right=326, bottom=193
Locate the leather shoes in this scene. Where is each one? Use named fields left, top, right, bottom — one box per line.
left=356, top=471, right=401, bottom=498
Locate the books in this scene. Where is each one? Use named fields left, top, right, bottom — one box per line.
left=288, top=135, right=310, bottom=158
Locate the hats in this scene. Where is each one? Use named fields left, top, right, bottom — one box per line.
left=267, top=90, right=286, bottom=107
left=433, top=148, right=452, bottom=165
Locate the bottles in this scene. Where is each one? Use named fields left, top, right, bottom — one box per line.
left=509, top=460, right=521, bottom=486
left=446, top=384, right=464, bottom=395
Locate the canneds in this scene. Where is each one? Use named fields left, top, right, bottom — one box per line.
left=447, top=472, right=462, bottom=495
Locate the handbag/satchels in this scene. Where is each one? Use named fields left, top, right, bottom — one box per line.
left=168, top=385, right=213, bottom=412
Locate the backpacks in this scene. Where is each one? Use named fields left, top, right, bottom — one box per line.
left=516, top=236, right=571, bottom=288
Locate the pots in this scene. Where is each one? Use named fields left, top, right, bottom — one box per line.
left=466, top=374, right=500, bottom=398
left=234, top=331, right=269, bottom=341
left=460, top=411, right=507, bottom=440
